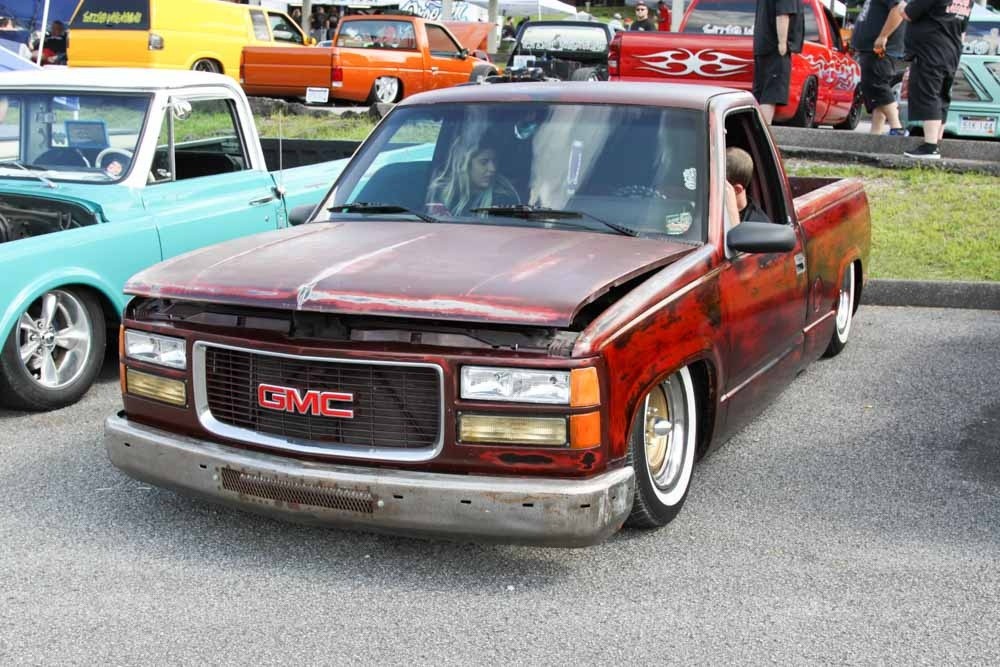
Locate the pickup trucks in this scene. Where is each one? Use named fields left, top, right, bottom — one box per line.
left=606, top=0, right=866, bottom=132
left=103, top=84, right=873, bottom=548
left=477, top=19, right=626, bottom=83
left=236, top=14, right=502, bottom=105
left=0, top=63, right=438, bottom=414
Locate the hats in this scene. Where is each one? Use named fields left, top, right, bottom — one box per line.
left=636, top=0, right=648, bottom=6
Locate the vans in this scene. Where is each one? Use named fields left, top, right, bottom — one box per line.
left=64, top=1, right=318, bottom=85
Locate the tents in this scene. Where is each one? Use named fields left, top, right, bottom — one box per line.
left=462, top=0, right=577, bottom=22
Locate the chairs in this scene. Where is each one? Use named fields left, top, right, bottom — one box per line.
left=32, top=148, right=241, bottom=180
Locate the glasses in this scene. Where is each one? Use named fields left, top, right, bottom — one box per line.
left=635, top=8, right=647, bottom=11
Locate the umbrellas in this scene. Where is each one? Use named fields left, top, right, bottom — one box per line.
left=0, top=0, right=79, bottom=64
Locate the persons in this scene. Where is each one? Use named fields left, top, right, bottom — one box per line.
left=501, top=15, right=530, bottom=40
left=421, top=122, right=513, bottom=219
left=724, top=146, right=769, bottom=228
left=901, top=0, right=974, bottom=157
left=653, top=0, right=670, bottom=32
left=290, top=4, right=339, bottom=41
left=631, top=1, right=657, bottom=33
left=752, top=0, right=805, bottom=126
left=30, top=20, right=68, bottom=67
left=849, top=0, right=911, bottom=137
left=608, top=11, right=626, bottom=40
left=0, top=15, right=14, bottom=28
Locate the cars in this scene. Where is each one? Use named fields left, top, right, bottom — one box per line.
left=889, top=53, right=1000, bottom=142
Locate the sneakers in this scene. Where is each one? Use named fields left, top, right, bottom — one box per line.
left=903, top=143, right=942, bottom=160
